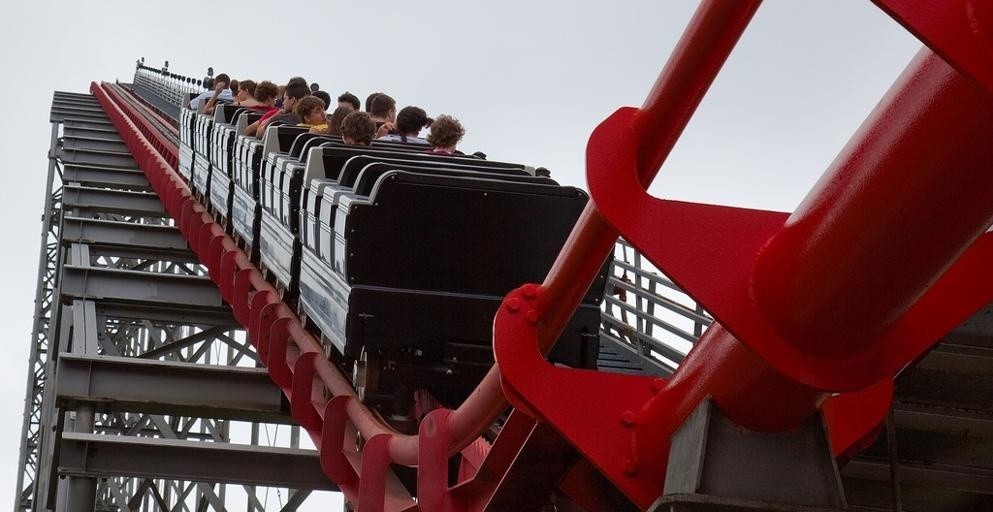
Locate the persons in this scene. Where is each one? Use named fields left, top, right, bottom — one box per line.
left=337, top=110, right=377, bottom=149
left=228, top=79, right=241, bottom=97
left=310, top=91, right=332, bottom=109
left=186, top=73, right=235, bottom=112
left=365, top=92, right=385, bottom=112
left=241, top=76, right=307, bottom=137
left=336, top=90, right=362, bottom=114
left=369, top=94, right=397, bottom=131
left=255, top=83, right=311, bottom=139
left=202, top=80, right=259, bottom=116
left=293, top=95, right=332, bottom=133
left=419, top=113, right=467, bottom=156
left=246, top=80, right=282, bottom=112
left=375, top=106, right=431, bottom=144
left=306, top=106, right=355, bottom=138
